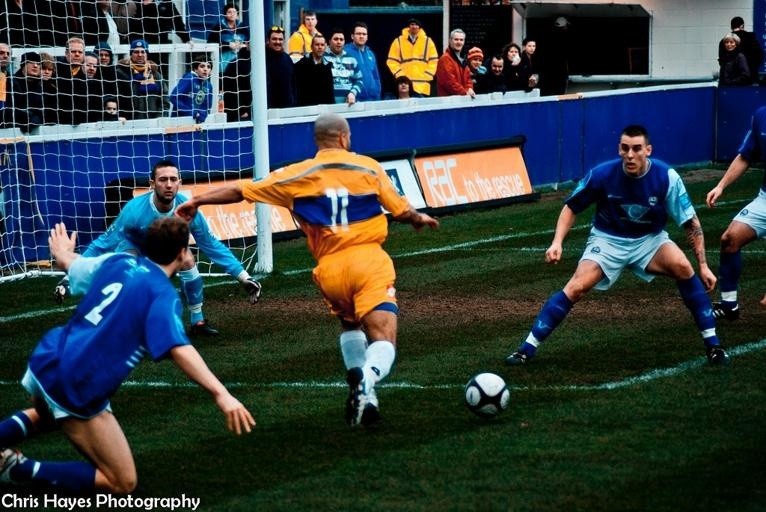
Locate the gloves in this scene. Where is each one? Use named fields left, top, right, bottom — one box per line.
left=236, top=270, right=262, bottom=306
left=53, top=275, right=75, bottom=305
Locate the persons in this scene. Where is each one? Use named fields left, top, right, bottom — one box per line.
left=501, top=123, right=731, bottom=365
left=704, top=104, right=766, bottom=323
left=0, top=216, right=258, bottom=497
left=169, top=113, right=439, bottom=432
left=716, top=33, right=751, bottom=86
left=54, top=161, right=263, bottom=336
left=727, top=12, right=764, bottom=84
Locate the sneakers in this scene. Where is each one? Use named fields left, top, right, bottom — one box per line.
left=344, top=365, right=370, bottom=428
left=0, top=448, right=30, bottom=487
left=356, top=389, right=382, bottom=417
left=706, top=341, right=731, bottom=366
left=503, top=349, right=530, bottom=367
left=696, top=300, right=742, bottom=322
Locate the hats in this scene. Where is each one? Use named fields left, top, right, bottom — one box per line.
left=466, top=46, right=484, bottom=61
left=21, top=52, right=42, bottom=65
left=130, top=39, right=149, bottom=56
left=555, top=17, right=570, bottom=28
left=408, top=16, right=422, bottom=27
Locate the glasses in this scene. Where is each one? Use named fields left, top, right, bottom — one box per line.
left=271, top=25, right=285, bottom=32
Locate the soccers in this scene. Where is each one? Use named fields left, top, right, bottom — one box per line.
left=464, top=373, right=511, bottom=417
